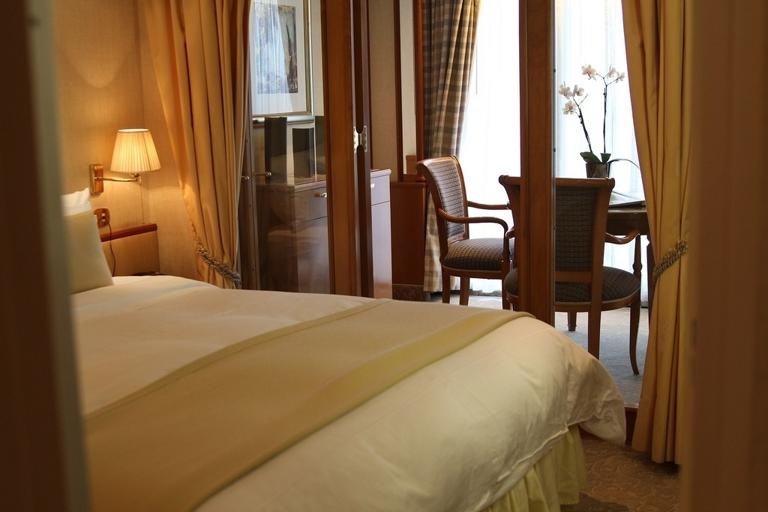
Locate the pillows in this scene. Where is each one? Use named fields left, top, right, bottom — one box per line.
left=60, top=185, right=114, bottom=295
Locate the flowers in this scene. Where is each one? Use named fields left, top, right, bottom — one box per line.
left=557, top=64, right=642, bottom=171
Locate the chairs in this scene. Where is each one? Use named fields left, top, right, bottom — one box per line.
left=497, top=173, right=640, bottom=377
left=415, top=155, right=512, bottom=308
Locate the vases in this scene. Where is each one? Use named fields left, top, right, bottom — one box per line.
left=585, top=160, right=611, bottom=178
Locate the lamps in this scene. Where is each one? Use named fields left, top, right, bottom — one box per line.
left=89, top=127, right=161, bottom=195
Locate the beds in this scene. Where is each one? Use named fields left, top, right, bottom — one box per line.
left=63, top=188, right=627, bottom=511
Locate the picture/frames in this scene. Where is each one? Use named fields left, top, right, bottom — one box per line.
left=250, top=1, right=314, bottom=127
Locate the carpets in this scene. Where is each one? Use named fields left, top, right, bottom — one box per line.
left=561, top=492, right=628, bottom=512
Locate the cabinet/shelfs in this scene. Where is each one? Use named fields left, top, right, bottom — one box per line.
left=256, top=168, right=392, bottom=299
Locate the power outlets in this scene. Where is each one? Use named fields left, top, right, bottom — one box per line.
left=94, top=208, right=110, bottom=228
